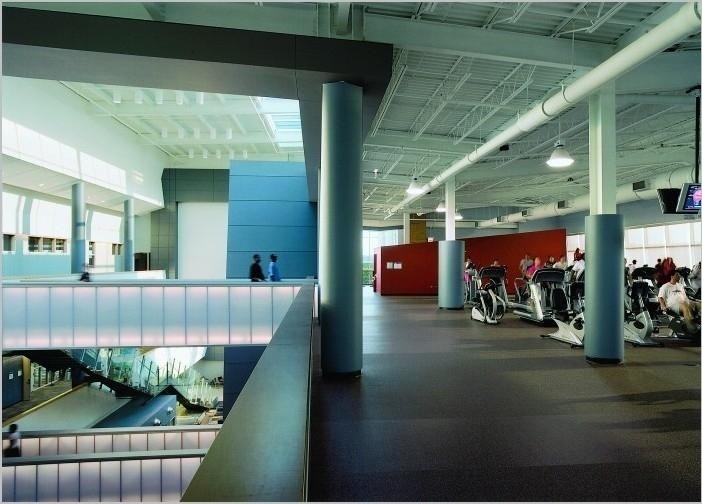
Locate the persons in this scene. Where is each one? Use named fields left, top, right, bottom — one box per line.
left=268, top=254, right=281, bottom=281
left=685, top=189, right=702, bottom=208
left=463, top=248, right=701, bottom=334
left=3, top=437, right=21, bottom=456
left=249, top=254, right=265, bottom=282
left=8, top=425, right=19, bottom=447
left=79, top=272, right=89, bottom=282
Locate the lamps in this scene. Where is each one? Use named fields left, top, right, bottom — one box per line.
left=406, top=174, right=424, bottom=196
left=435, top=187, right=464, bottom=221
left=546, top=118, right=575, bottom=169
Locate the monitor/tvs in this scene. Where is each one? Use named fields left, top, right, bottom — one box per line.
left=676, top=182, right=702, bottom=214
left=655, top=188, right=699, bottom=215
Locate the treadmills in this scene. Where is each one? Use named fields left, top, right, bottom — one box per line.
left=468, top=265, right=513, bottom=313
left=514, top=268, right=569, bottom=326
